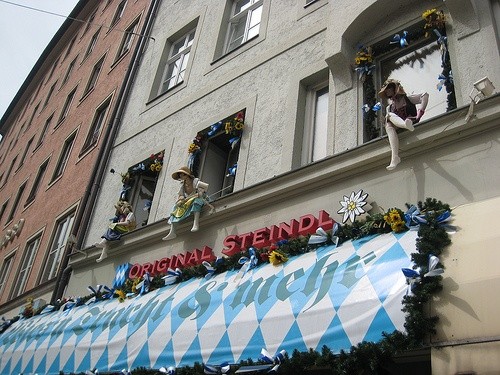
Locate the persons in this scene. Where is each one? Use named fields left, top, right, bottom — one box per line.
left=161, top=166, right=209, bottom=240
left=93, top=201, right=136, bottom=262
left=377, top=77, right=428, bottom=172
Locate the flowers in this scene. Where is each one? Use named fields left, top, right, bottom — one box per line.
left=384, top=209, right=407, bottom=233
left=267, top=251, right=288, bottom=265
left=225, top=118, right=243, bottom=134
left=150, top=160, right=162, bottom=171
left=355, top=46, right=373, bottom=66
left=337, top=189, right=372, bottom=226
left=114, top=275, right=142, bottom=301
left=187, top=143, right=202, bottom=155
left=422, top=7, right=446, bottom=37
